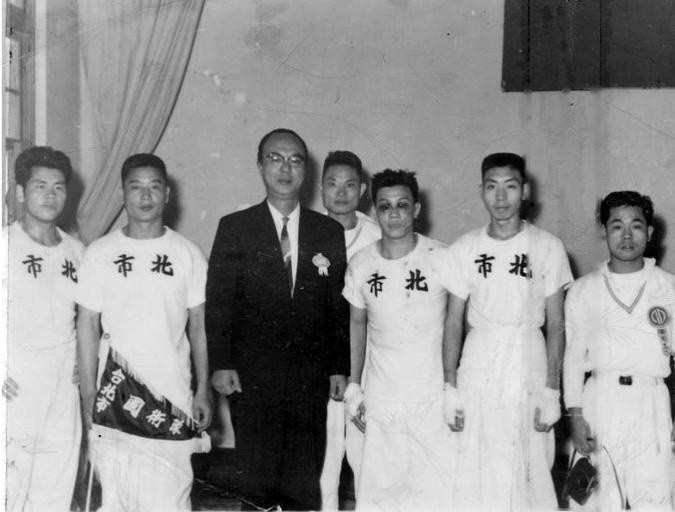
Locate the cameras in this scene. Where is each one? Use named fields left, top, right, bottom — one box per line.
left=566, top=458, right=599, bottom=506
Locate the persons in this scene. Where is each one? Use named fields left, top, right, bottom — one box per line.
left=318, top=150, right=383, bottom=512
left=341, top=169, right=453, bottom=512
left=562, top=191, right=675, bottom=511
left=73, top=153, right=208, bottom=512
left=440, top=153, right=576, bottom=512
left=205, top=128, right=350, bottom=512
left=1, top=146, right=83, bottom=512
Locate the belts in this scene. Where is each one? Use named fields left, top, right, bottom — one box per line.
left=591, top=369, right=666, bottom=387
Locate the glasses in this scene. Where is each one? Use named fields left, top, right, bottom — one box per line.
left=260, top=153, right=306, bottom=168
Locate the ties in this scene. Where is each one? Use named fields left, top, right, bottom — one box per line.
left=279, top=216, right=293, bottom=293
